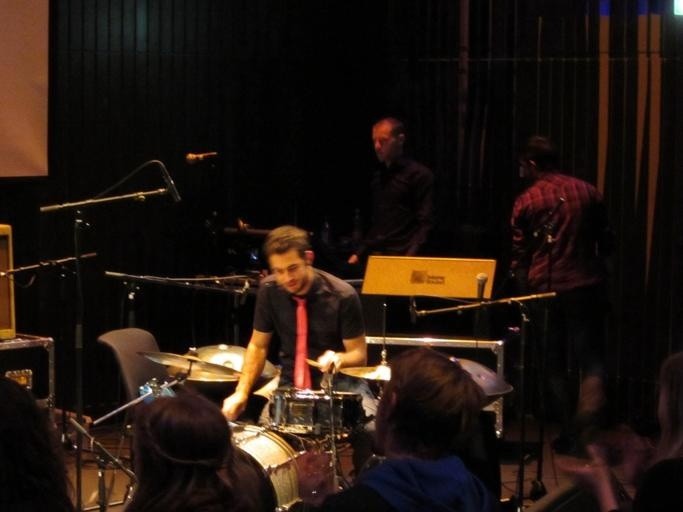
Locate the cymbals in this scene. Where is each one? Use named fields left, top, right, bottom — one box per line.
left=187, top=344, right=281, bottom=378
left=137, top=348, right=239, bottom=375
left=444, top=359, right=514, bottom=395
left=341, top=366, right=393, bottom=383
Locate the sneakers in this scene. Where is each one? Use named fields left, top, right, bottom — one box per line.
left=551, top=411, right=599, bottom=457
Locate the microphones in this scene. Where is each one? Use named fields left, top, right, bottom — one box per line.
left=474, top=272, right=487, bottom=320
left=187, top=151, right=218, bottom=165
left=161, top=168, right=182, bottom=201
left=321, top=364, right=335, bottom=392
left=222, top=275, right=255, bottom=292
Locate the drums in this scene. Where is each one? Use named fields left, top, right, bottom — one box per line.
left=226, top=425, right=302, bottom=511
left=273, top=388, right=364, bottom=438
left=272, top=428, right=348, bottom=502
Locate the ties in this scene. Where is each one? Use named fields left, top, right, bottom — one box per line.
left=294, top=298, right=312, bottom=390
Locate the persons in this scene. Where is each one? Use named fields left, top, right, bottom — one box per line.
left=221, top=226, right=368, bottom=424
left=288, top=348, right=507, bottom=512
left=0, top=377, right=77, bottom=511
left=347, top=116, right=435, bottom=267
left=122, top=395, right=279, bottom=512
left=510, top=135, right=609, bottom=458
left=555, top=351, right=683, bottom=511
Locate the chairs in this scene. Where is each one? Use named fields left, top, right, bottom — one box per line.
left=96, top=328, right=165, bottom=416
